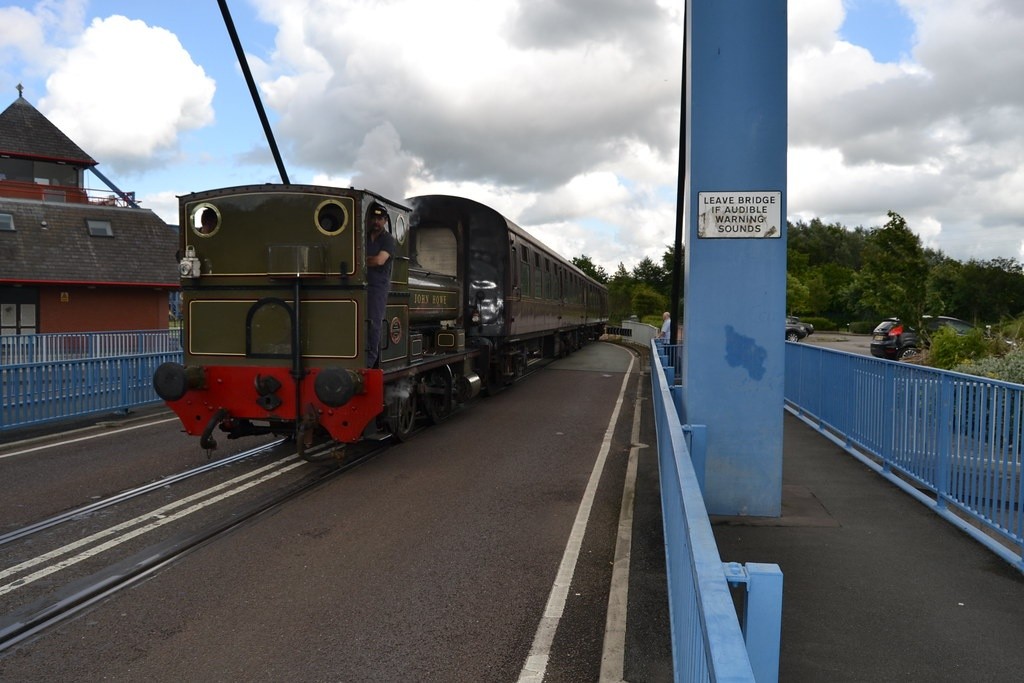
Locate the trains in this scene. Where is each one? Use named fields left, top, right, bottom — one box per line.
left=152, top=183, right=612, bottom=458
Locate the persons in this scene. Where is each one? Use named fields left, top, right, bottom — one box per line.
left=365, top=206, right=396, bottom=368
left=658, top=312, right=671, bottom=355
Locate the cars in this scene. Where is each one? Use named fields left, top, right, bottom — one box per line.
left=785, top=315, right=815, bottom=343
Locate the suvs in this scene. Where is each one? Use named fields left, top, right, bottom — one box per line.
left=869, top=315, right=1017, bottom=361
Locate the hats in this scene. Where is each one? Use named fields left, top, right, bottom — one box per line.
left=371, top=208, right=386, bottom=218
